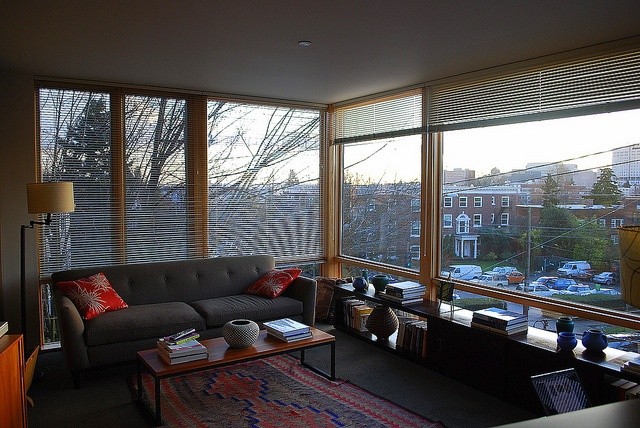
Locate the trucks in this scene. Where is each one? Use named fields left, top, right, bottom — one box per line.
left=594, top=272, right=620, bottom=285
left=557, top=261, right=591, bottom=278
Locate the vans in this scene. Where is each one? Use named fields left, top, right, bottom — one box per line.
left=560, top=285, right=591, bottom=296
left=442, top=265, right=482, bottom=280
left=516, top=284, right=559, bottom=296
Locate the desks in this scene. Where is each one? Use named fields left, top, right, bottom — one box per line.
left=135, top=323, right=336, bottom=426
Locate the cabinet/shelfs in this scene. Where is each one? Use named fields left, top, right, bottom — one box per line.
left=1, top=334, right=29, bottom=428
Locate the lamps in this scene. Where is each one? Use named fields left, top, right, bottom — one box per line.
left=22, top=181, right=75, bottom=361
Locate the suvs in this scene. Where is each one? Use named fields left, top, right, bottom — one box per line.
left=532, top=277, right=559, bottom=287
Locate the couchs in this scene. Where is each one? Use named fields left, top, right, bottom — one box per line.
left=49, top=254, right=318, bottom=376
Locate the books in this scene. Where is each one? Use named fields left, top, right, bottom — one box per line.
left=470, top=306, right=529, bottom=336
left=340, top=295, right=375, bottom=333
left=378, top=280, right=428, bottom=305
left=619, top=355, right=640, bottom=376
left=610, top=378, right=639, bottom=400
left=262, top=317, right=314, bottom=344
left=395, top=319, right=428, bottom=357
left=0, top=320, right=10, bottom=339
left=156, top=331, right=210, bottom=366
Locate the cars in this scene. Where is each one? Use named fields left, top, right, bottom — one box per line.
left=495, top=267, right=516, bottom=277
left=504, top=269, right=516, bottom=275
left=484, top=267, right=501, bottom=275
left=506, top=272, right=524, bottom=284
left=547, top=278, right=577, bottom=290
left=592, top=288, right=618, bottom=295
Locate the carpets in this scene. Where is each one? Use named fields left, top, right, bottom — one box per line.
left=122, top=352, right=445, bottom=428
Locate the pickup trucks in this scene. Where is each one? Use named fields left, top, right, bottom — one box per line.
left=478, top=275, right=508, bottom=288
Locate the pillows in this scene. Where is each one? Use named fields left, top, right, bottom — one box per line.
left=55, top=271, right=129, bottom=322
left=241, top=268, right=304, bottom=300
